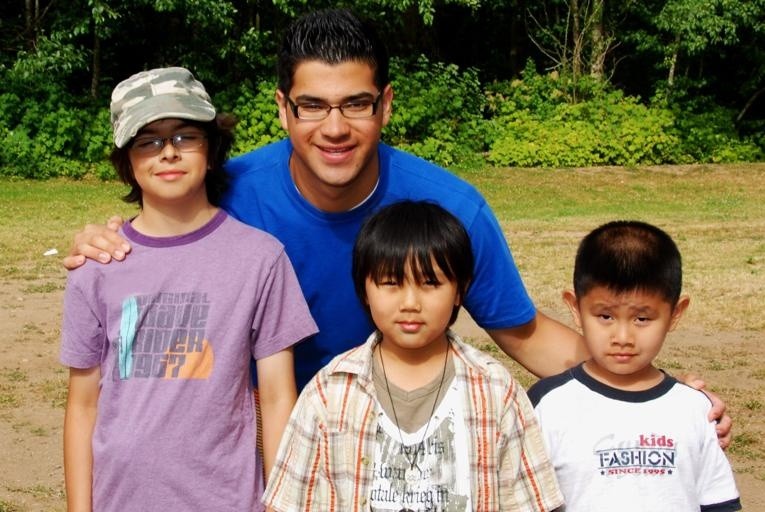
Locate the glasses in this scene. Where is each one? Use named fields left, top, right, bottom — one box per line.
left=132, top=134, right=209, bottom=153
left=285, top=90, right=383, bottom=120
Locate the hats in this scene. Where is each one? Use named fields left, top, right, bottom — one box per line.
left=110, top=67, right=216, bottom=149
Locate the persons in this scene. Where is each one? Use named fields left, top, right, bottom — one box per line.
left=56, top=67, right=315, bottom=512
left=522, top=220, right=748, bottom=512
left=255, top=197, right=568, bottom=511
left=63, top=5, right=734, bottom=455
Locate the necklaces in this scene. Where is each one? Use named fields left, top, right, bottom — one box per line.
left=376, top=337, right=450, bottom=483
